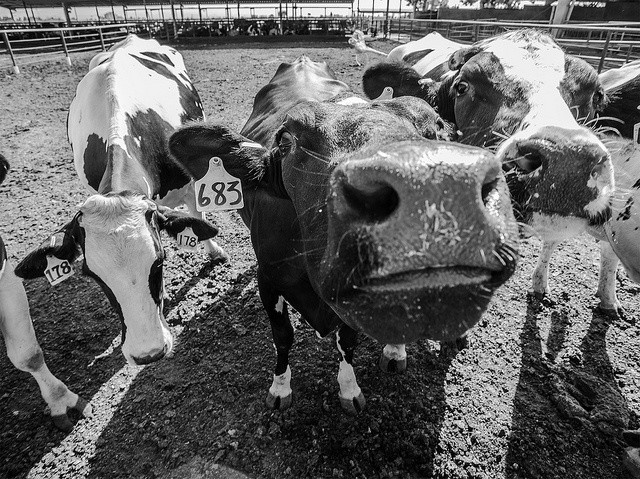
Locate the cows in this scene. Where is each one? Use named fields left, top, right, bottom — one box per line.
left=531, top=59, right=640, bottom=319
left=0, top=153, right=94, bottom=432
left=163, top=55, right=527, bottom=415
left=13, top=34, right=231, bottom=369
left=379, top=27, right=624, bottom=375
